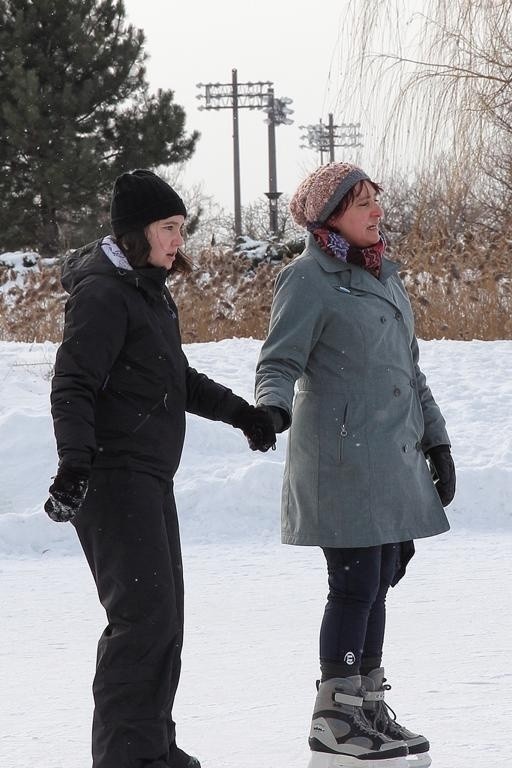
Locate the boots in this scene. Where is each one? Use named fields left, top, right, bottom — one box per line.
left=308, top=667, right=430, bottom=762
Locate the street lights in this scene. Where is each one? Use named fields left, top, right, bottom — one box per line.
left=264, top=191, right=283, bottom=231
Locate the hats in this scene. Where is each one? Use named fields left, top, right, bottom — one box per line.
left=289, top=162, right=370, bottom=229
left=110, top=169, right=187, bottom=238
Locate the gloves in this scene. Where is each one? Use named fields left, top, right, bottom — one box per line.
left=426, top=444, right=457, bottom=509
left=44, top=470, right=90, bottom=523
left=233, top=405, right=283, bottom=453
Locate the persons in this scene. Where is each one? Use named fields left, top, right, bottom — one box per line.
left=50, top=169, right=275, bottom=768
left=248, top=161, right=456, bottom=761
